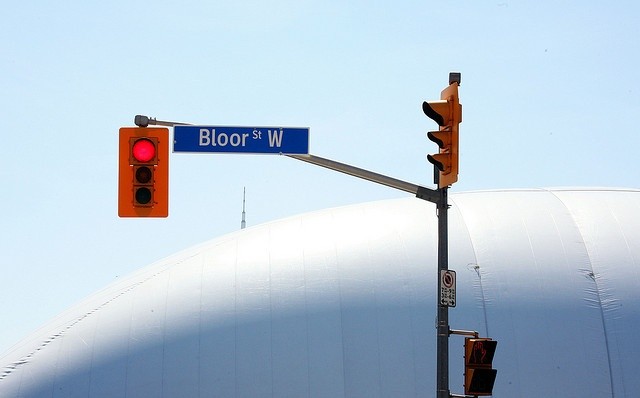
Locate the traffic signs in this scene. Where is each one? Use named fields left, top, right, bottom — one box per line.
left=176, top=127, right=307, bottom=152
left=440, top=269, right=456, bottom=306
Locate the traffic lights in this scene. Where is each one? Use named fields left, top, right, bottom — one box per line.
left=423, top=83, right=458, bottom=187
left=464, top=337, right=497, bottom=396
left=118, top=129, right=167, bottom=216
